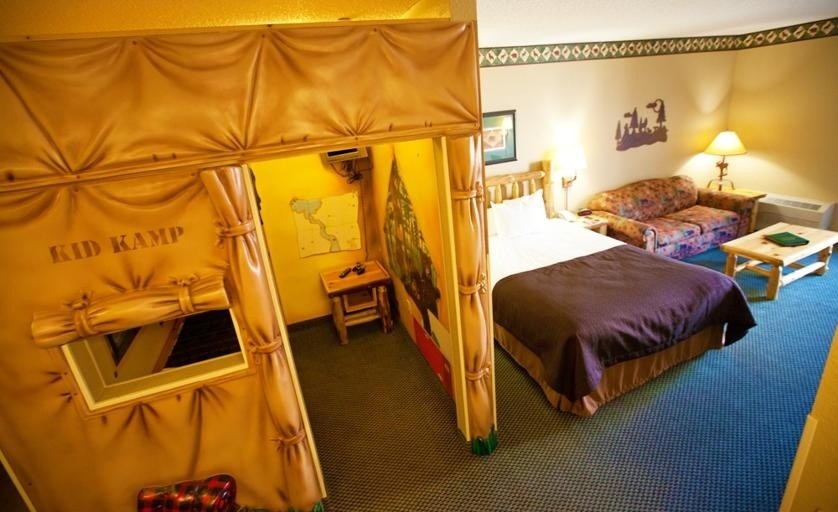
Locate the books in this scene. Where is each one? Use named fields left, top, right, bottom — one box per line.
left=765, top=231, right=810, bottom=246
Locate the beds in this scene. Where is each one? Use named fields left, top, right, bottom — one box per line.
left=486, top=160, right=757, bottom=417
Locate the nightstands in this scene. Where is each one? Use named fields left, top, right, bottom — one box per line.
left=566, top=211, right=608, bottom=235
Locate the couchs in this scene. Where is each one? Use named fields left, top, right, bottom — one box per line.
left=588, top=175, right=754, bottom=260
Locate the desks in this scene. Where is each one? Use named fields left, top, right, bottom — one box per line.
left=712, top=187, right=767, bottom=233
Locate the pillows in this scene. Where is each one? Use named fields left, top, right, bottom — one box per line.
left=485, top=188, right=548, bottom=238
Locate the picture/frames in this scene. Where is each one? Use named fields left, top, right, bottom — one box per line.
left=483, top=110, right=517, bottom=165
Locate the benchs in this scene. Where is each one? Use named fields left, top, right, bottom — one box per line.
left=758, top=192, right=834, bottom=229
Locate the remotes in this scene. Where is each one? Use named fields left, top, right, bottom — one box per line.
left=339, top=268, right=350, bottom=278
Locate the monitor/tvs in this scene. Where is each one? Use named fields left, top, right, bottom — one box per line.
left=326, top=146, right=368, bottom=164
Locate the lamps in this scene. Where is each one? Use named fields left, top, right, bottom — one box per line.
left=703, top=131, right=747, bottom=191
left=554, top=137, right=585, bottom=221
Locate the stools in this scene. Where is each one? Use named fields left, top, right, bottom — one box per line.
left=319, top=260, right=393, bottom=345
